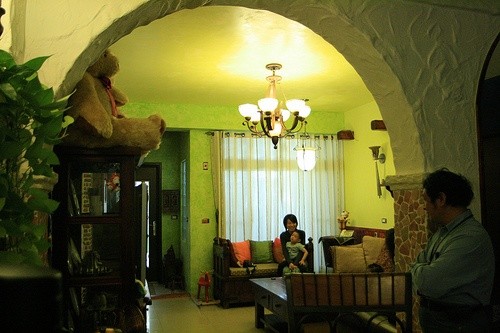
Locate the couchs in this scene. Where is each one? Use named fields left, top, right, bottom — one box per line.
left=213, top=233, right=286, bottom=307
left=288, top=274, right=413, bottom=333
left=321, top=219, right=394, bottom=273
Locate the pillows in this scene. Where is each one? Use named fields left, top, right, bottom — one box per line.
left=231, top=238, right=282, bottom=264
left=330, top=236, right=386, bottom=272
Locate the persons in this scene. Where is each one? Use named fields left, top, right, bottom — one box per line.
left=410, top=167, right=494, bottom=333
left=278, top=232, right=308, bottom=277
left=280, top=214, right=305, bottom=273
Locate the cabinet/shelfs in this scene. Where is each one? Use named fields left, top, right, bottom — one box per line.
left=63, top=149, right=128, bottom=306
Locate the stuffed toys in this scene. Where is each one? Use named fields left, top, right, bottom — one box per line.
left=107, top=172, right=120, bottom=192
left=64, top=50, right=166, bottom=158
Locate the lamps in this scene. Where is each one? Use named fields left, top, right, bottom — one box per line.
left=238, top=63, right=312, bottom=149
left=295, top=121, right=317, bottom=172
left=369, top=145, right=386, bottom=198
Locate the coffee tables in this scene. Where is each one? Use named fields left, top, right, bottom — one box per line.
left=249, top=277, right=289, bottom=333
